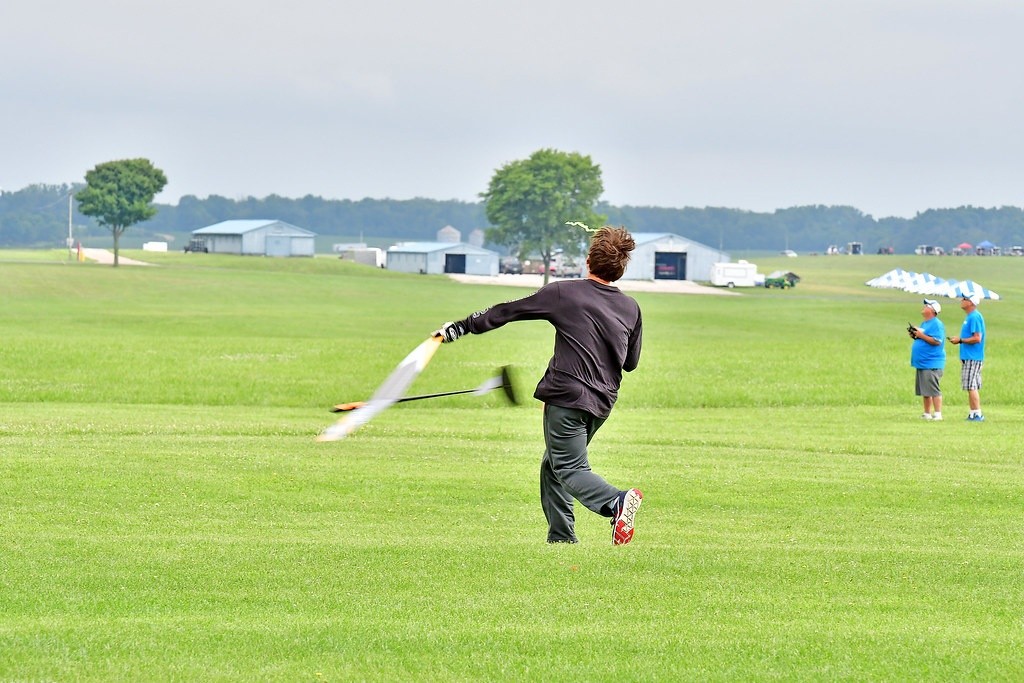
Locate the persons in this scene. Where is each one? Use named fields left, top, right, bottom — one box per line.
left=909, top=298, right=946, bottom=420
left=430, top=227, right=642, bottom=547
left=949, top=292, right=985, bottom=422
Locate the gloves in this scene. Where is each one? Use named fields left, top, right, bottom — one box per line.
left=430, top=316, right=470, bottom=343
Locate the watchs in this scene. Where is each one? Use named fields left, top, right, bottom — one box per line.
left=959, top=338, right=963, bottom=344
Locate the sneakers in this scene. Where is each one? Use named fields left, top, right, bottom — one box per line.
left=971, top=413, right=984, bottom=422
left=610, top=487, right=644, bottom=546
left=966, top=414, right=973, bottom=420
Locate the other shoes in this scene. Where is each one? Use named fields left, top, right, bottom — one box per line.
left=931, top=412, right=942, bottom=420
left=921, top=413, right=932, bottom=420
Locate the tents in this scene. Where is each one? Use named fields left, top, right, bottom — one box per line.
left=978, top=240, right=996, bottom=248
left=959, top=242, right=972, bottom=249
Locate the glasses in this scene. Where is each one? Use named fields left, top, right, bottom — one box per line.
left=964, top=297, right=967, bottom=300
left=925, top=304, right=935, bottom=312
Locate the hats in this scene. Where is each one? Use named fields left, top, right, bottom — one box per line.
left=962, top=292, right=980, bottom=306
left=924, top=299, right=941, bottom=315
